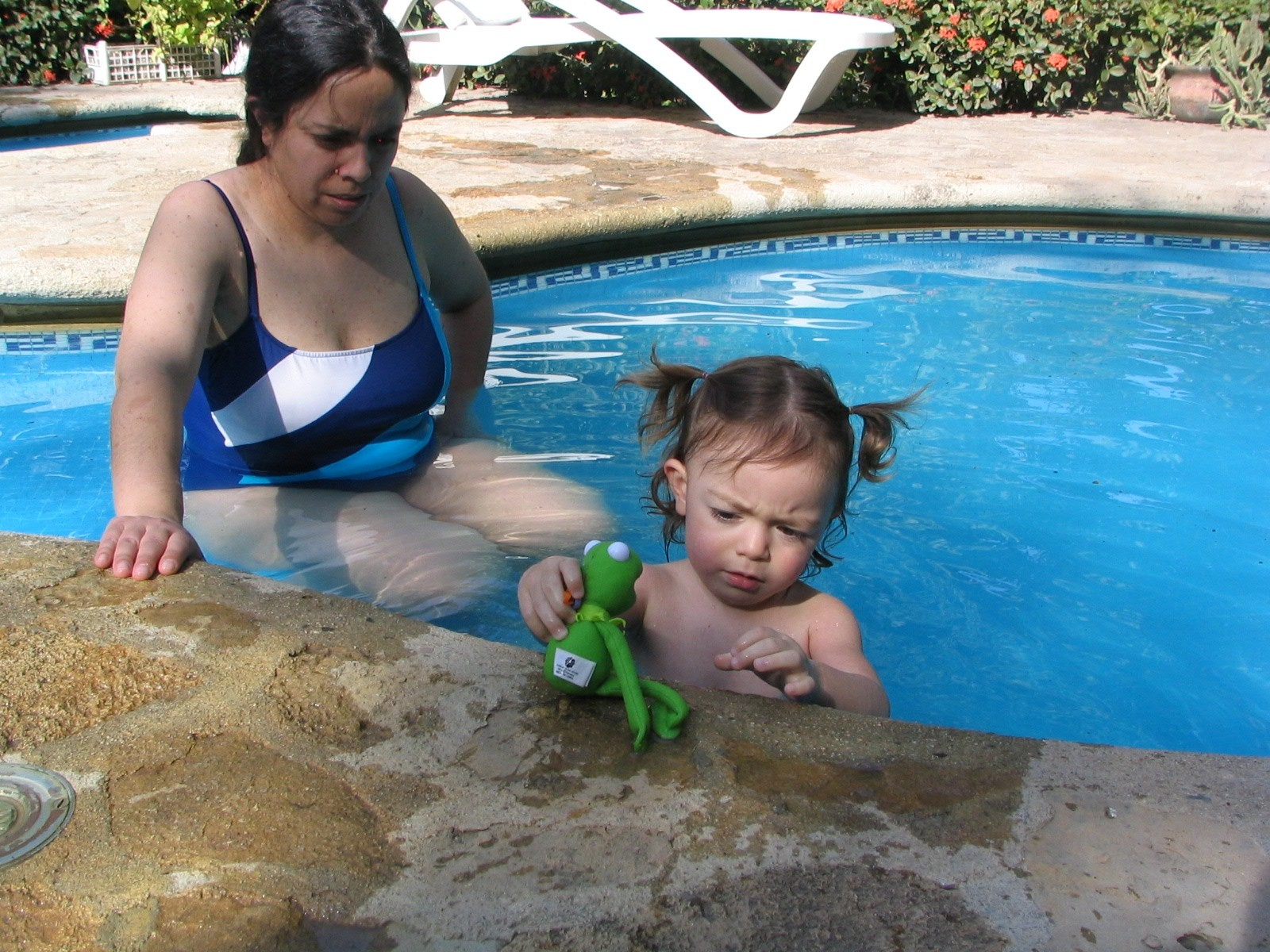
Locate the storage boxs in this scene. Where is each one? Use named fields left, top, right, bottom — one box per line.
left=83, top=41, right=222, bottom=86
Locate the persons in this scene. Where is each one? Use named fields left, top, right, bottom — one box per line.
left=92, top=0, right=614, bottom=626
left=517, top=343, right=931, bottom=717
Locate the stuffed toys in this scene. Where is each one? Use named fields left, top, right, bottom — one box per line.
left=542, top=539, right=685, bottom=750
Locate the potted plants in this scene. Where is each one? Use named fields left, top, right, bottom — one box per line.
left=1123, top=19, right=1270, bottom=131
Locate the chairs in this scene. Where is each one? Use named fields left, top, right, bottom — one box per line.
left=384, top=0, right=896, bottom=138
